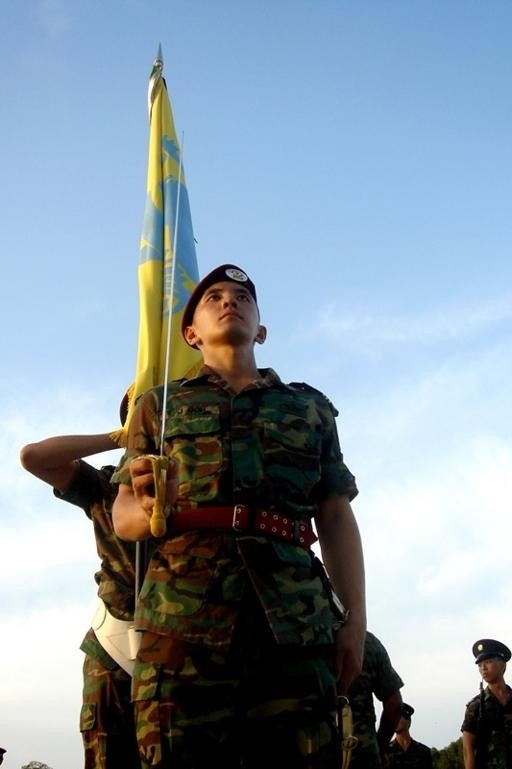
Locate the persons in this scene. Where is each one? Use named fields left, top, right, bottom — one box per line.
left=111, top=265, right=367, bottom=769
left=462, top=640, right=511, bottom=769
left=20, top=384, right=145, bottom=768
left=334, top=628, right=432, bottom=768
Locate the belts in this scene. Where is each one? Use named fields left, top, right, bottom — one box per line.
left=169, top=504, right=318, bottom=550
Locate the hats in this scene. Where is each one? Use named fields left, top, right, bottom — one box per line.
left=119, top=392, right=128, bottom=427
left=181, top=263, right=257, bottom=350
left=472, top=639, right=512, bottom=664
left=402, top=703, right=415, bottom=717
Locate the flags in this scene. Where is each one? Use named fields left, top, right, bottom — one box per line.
left=110, top=61, right=201, bottom=451
